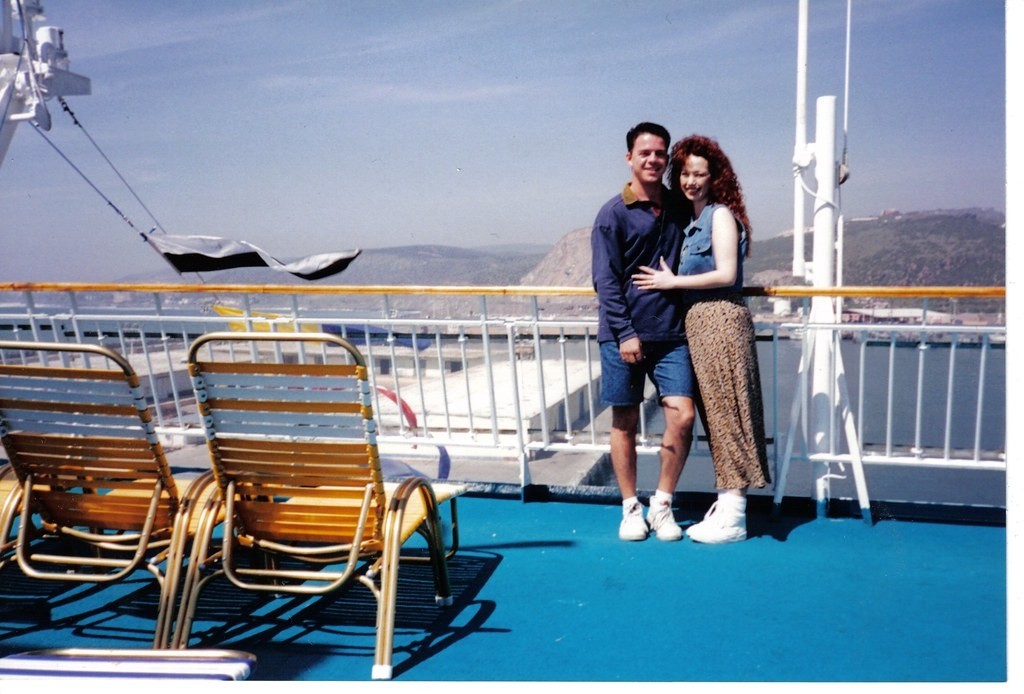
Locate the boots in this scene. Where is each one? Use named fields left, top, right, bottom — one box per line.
left=685, top=492, right=748, bottom=544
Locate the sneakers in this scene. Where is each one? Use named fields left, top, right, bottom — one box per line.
left=619, top=501, right=648, bottom=540
left=646, top=496, right=682, bottom=541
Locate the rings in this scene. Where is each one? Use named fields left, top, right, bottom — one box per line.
left=650, top=281, right=653, bottom=285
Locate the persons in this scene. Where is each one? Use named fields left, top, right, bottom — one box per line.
left=591, top=121, right=694, bottom=541
left=632, top=134, right=771, bottom=543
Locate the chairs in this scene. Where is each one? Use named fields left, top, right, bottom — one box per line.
left=0, top=332, right=467, bottom=681
